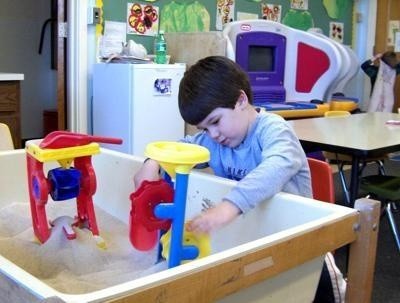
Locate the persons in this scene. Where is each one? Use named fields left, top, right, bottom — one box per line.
left=361, top=51, right=400, bottom=112
left=132, top=55, right=347, bottom=303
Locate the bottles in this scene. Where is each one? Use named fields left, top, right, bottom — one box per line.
left=155, top=31, right=167, bottom=64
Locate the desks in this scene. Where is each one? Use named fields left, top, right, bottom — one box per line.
left=287, top=110, right=400, bottom=207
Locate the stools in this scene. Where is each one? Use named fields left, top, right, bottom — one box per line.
left=359, top=172, right=400, bottom=254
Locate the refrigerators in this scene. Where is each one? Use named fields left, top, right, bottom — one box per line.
left=92, top=63, right=186, bottom=157
